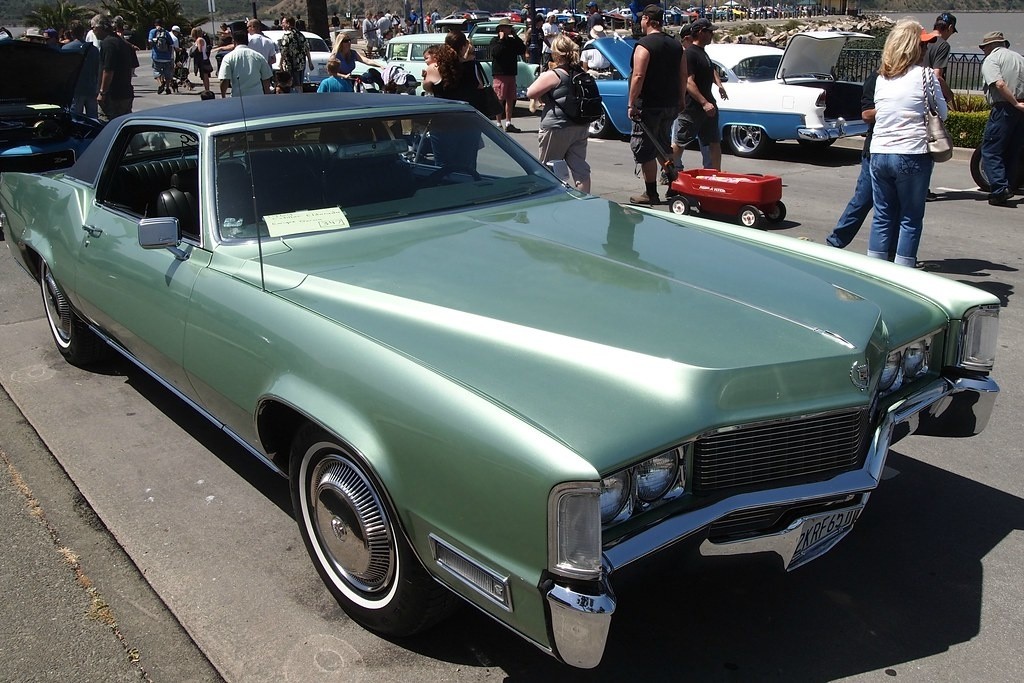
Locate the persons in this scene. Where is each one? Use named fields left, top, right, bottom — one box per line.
left=317, top=2, right=613, bottom=194
left=827, top=12, right=957, bottom=269
left=41, top=14, right=140, bottom=122
left=148, top=12, right=314, bottom=101
left=979, top=31, right=1024, bottom=205
left=627, top=0, right=828, bottom=204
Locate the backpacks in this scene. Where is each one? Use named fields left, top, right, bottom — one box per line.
left=546, top=65, right=602, bottom=122
left=520, top=9, right=528, bottom=19
left=152, top=28, right=168, bottom=52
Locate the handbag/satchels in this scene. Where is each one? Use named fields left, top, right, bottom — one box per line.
left=475, top=61, right=503, bottom=116
left=202, top=59, right=213, bottom=73
left=354, top=24, right=360, bottom=29
left=922, top=66, right=952, bottom=162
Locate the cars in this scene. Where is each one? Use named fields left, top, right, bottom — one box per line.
left=435, top=8, right=633, bottom=34
left=582, top=30, right=873, bottom=157
left=348, top=23, right=540, bottom=99
left=663, top=7, right=799, bottom=24
left=1, top=37, right=108, bottom=176
left=217, top=31, right=331, bottom=87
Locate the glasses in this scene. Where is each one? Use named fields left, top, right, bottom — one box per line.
left=246, top=25, right=255, bottom=29
left=91, top=26, right=99, bottom=30
left=343, top=39, right=351, bottom=43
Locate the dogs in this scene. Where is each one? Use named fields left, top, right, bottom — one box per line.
left=530, top=62, right=558, bottom=112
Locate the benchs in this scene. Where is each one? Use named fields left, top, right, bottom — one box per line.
left=109, top=142, right=339, bottom=234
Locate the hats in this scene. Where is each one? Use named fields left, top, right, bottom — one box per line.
left=152, top=19, right=180, bottom=32
left=547, top=2, right=607, bottom=40
left=921, top=29, right=941, bottom=43
left=979, top=32, right=1010, bottom=50
left=679, top=19, right=719, bottom=39
left=937, top=13, right=958, bottom=33
left=636, top=5, right=663, bottom=21
left=26, top=29, right=50, bottom=38
left=496, top=18, right=514, bottom=31
left=524, top=4, right=530, bottom=7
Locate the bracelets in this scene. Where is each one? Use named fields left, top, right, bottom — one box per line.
left=99, top=89, right=107, bottom=95
left=627, top=104, right=636, bottom=108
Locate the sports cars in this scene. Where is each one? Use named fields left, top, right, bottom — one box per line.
left=0, top=93, right=1003, bottom=670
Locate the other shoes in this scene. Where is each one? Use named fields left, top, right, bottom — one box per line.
left=630, top=176, right=672, bottom=205
left=989, top=188, right=1014, bottom=205
left=914, top=262, right=925, bottom=270
left=157, top=85, right=171, bottom=94
left=505, top=125, right=521, bottom=132
left=926, top=189, right=937, bottom=200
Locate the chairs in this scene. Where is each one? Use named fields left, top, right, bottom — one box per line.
left=320, top=155, right=417, bottom=209
left=170, top=162, right=246, bottom=235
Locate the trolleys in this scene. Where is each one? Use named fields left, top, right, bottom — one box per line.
left=631, top=109, right=787, bottom=227
